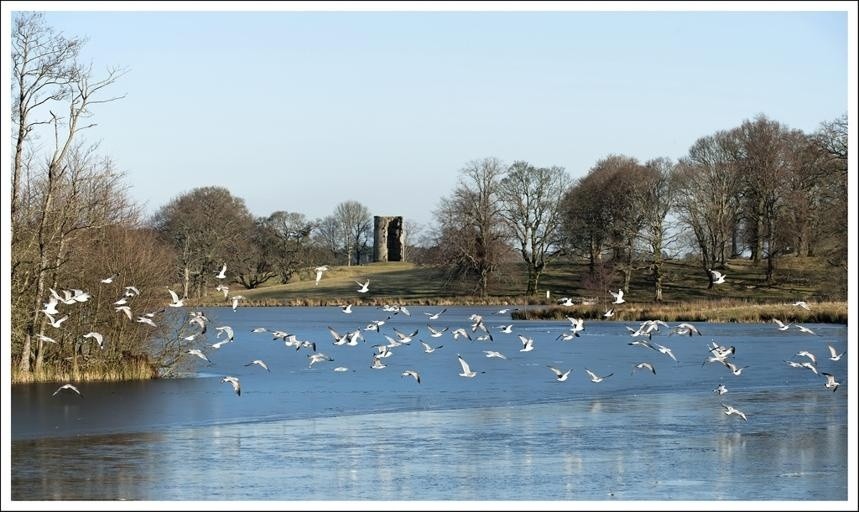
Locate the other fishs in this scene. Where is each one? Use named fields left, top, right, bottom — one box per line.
left=713, top=382, right=731, bottom=396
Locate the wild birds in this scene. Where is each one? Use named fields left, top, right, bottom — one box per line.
left=54, top=382, right=82, bottom=397
left=112, top=287, right=166, bottom=327
left=167, top=286, right=190, bottom=311
left=215, top=285, right=247, bottom=311
left=773, top=299, right=847, bottom=393
left=182, top=311, right=213, bottom=364
left=518, top=335, right=535, bottom=353
left=314, top=263, right=328, bottom=288
left=219, top=374, right=242, bottom=397
left=554, top=287, right=626, bottom=341
left=34, top=286, right=109, bottom=350
left=355, top=278, right=370, bottom=293
left=703, top=341, right=749, bottom=378
left=547, top=367, right=616, bottom=386
left=100, top=273, right=117, bottom=284
left=216, top=263, right=228, bottom=280
left=625, top=321, right=702, bottom=374
left=721, top=402, right=748, bottom=423
left=213, top=326, right=236, bottom=349
left=711, top=269, right=727, bottom=284
left=329, top=303, right=512, bottom=386
left=242, top=326, right=334, bottom=372
